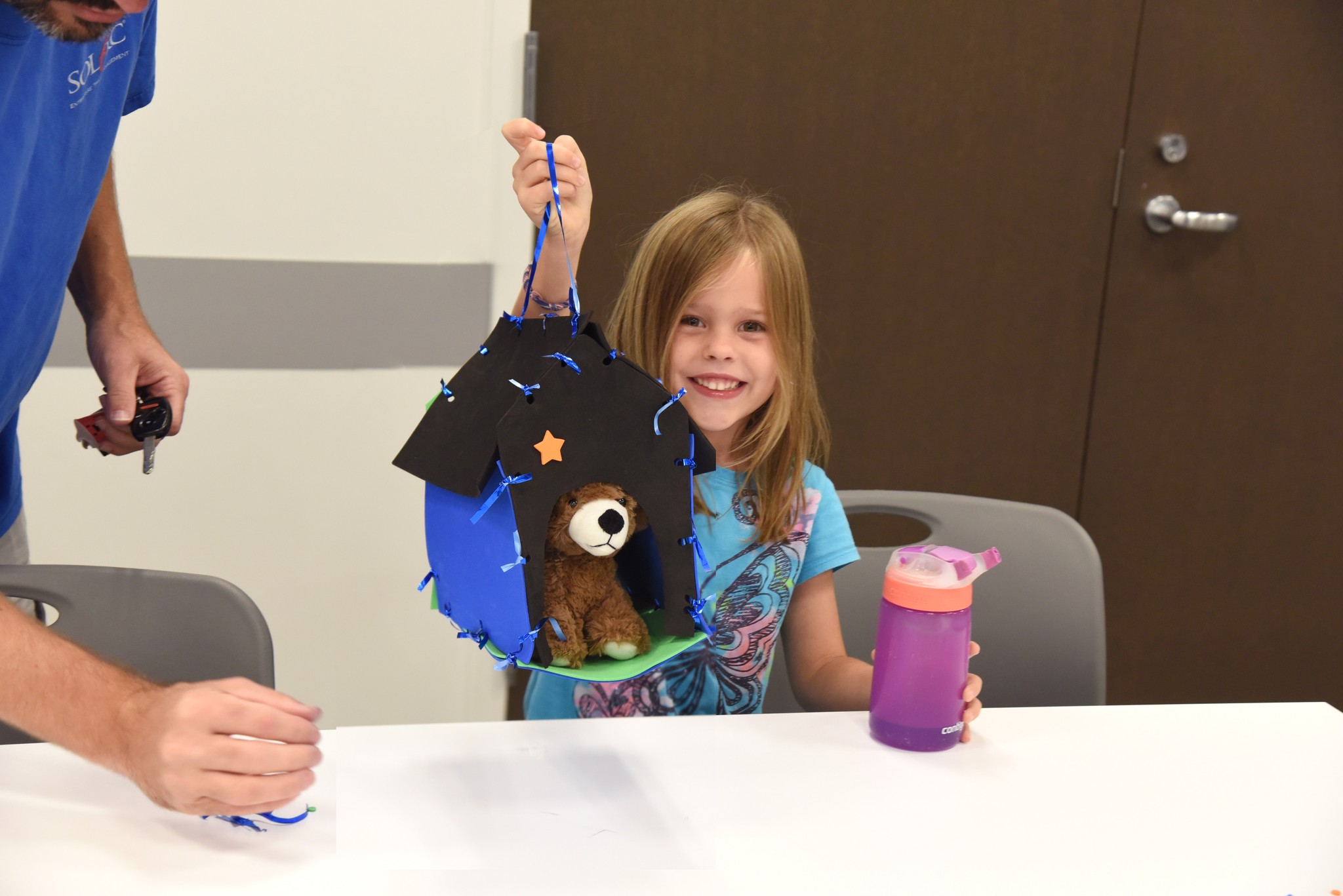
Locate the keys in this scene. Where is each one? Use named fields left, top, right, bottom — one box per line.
left=126, top=385, right=173, bottom=475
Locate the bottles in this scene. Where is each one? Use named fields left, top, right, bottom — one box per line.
left=868, top=542, right=1002, bottom=752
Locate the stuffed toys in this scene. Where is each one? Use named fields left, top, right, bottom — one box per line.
left=544, top=484, right=650, bottom=666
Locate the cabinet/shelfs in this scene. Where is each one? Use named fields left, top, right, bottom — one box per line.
left=527, top=0, right=1343, bottom=713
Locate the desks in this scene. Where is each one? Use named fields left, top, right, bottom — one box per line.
left=0, top=696, right=1343, bottom=896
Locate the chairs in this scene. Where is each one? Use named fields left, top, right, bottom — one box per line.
left=755, top=486, right=1118, bottom=715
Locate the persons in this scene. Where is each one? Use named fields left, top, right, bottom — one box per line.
left=0, top=0, right=323, bottom=818
left=501, top=118, right=984, bottom=743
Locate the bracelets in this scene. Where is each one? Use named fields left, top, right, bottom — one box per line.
left=523, top=264, right=579, bottom=311
